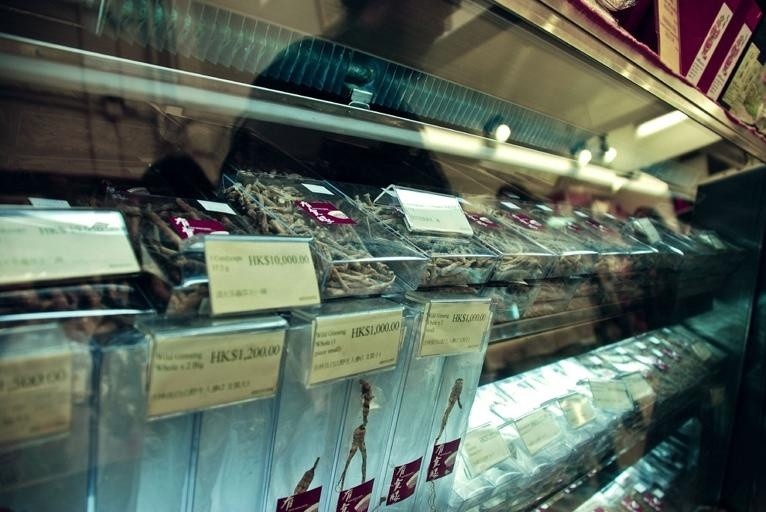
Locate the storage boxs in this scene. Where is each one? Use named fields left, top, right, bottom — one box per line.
left=224, top=174, right=426, bottom=298
left=471, top=212, right=555, bottom=282
left=351, top=198, right=497, bottom=287
left=630, top=233, right=684, bottom=273
left=569, top=217, right=657, bottom=274
left=660, top=233, right=698, bottom=271
left=534, top=214, right=629, bottom=276
left=497, top=213, right=598, bottom=277
